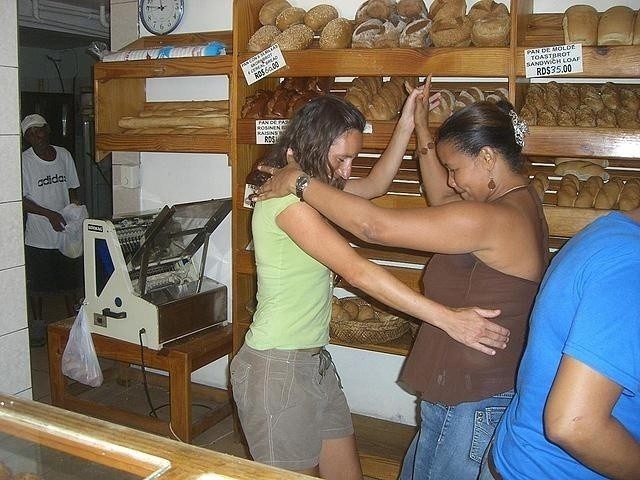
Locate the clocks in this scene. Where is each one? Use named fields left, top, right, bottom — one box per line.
left=139, top=0, right=184, bottom=37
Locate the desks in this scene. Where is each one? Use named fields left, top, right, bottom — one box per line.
left=46, top=311, right=237, bottom=451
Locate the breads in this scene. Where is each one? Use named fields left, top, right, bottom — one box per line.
left=240, top=74, right=509, bottom=123
left=530, top=157, right=639, bottom=213
left=330, top=299, right=405, bottom=324
left=563, top=5, right=600, bottom=47
left=597, top=5, right=634, bottom=46
left=247, top=0, right=510, bottom=52
left=518, top=80, right=640, bottom=132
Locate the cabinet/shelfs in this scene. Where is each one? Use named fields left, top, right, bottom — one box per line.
left=93, top=29, right=235, bottom=166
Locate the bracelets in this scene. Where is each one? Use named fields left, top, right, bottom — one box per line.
left=296, top=173, right=310, bottom=201
left=413, top=137, right=436, bottom=157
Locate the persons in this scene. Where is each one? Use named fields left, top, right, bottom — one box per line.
left=244, top=71, right=549, bottom=480
left=474, top=198, right=640, bottom=480
left=224, top=81, right=513, bottom=480
left=21, top=112, right=84, bottom=351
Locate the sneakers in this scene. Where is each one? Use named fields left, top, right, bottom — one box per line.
left=30, top=319, right=47, bottom=347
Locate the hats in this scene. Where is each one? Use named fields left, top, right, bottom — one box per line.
left=21, top=114, right=47, bottom=136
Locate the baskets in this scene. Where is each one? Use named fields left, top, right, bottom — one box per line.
left=331, top=295, right=421, bottom=346
